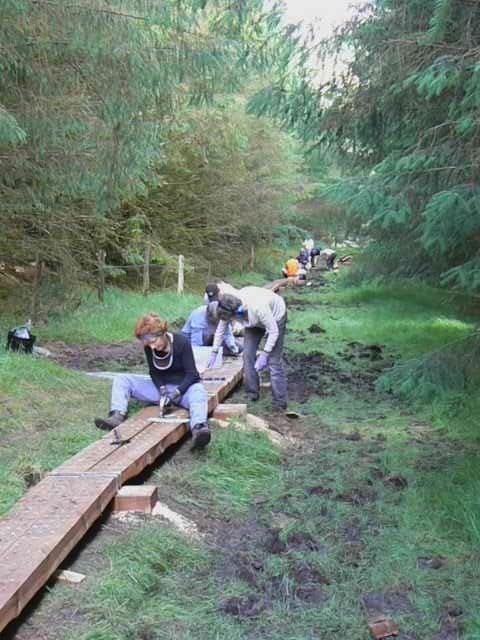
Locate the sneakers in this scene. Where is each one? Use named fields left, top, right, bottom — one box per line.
left=248, top=392, right=258, bottom=401
left=270, top=407, right=287, bottom=416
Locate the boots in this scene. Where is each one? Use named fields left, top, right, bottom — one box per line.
left=192, top=423, right=210, bottom=448
left=96, top=410, right=128, bottom=431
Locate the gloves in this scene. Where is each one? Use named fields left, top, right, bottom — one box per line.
left=160, top=386, right=181, bottom=407
left=208, top=352, right=217, bottom=368
left=254, top=351, right=269, bottom=372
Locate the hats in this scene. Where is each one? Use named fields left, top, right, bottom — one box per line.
left=206, top=284, right=219, bottom=296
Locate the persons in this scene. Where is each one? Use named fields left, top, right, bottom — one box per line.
left=94, top=312, right=211, bottom=452
left=207, top=285, right=288, bottom=411
left=180, top=301, right=244, bottom=355
left=280, top=235, right=337, bottom=277
left=204, top=280, right=244, bottom=335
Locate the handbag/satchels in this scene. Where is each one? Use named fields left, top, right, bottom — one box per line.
left=7, top=326, right=36, bottom=353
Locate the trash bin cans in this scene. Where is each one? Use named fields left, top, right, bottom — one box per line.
left=7, top=331, right=36, bottom=354
